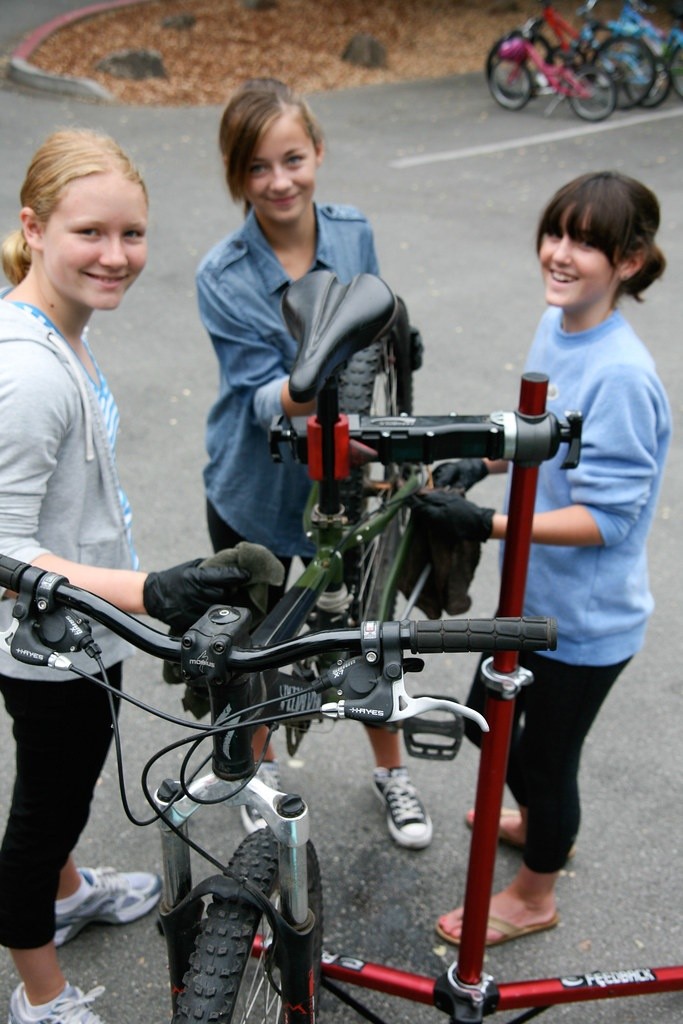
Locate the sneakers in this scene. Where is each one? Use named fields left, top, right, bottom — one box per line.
left=373, top=767, right=433, bottom=847
left=6, top=980, right=106, bottom=1024
left=241, top=754, right=282, bottom=833
left=53, top=866, right=160, bottom=950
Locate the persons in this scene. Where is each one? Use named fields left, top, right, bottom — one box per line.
left=194, top=80, right=435, bottom=860
left=435, top=168, right=672, bottom=947
left=0, top=125, right=255, bottom=1024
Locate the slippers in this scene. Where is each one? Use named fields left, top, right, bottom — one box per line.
left=439, top=909, right=560, bottom=944
left=467, top=806, right=579, bottom=860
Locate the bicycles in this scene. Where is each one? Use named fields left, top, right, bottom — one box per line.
left=0, top=270, right=585, bottom=1024
left=576, top=0, right=672, bottom=109
left=621, top=1, right=682, bottom=99
left=488, top=17, right=618, bottom=123
left=521, top=0, right=656, bottom=109
